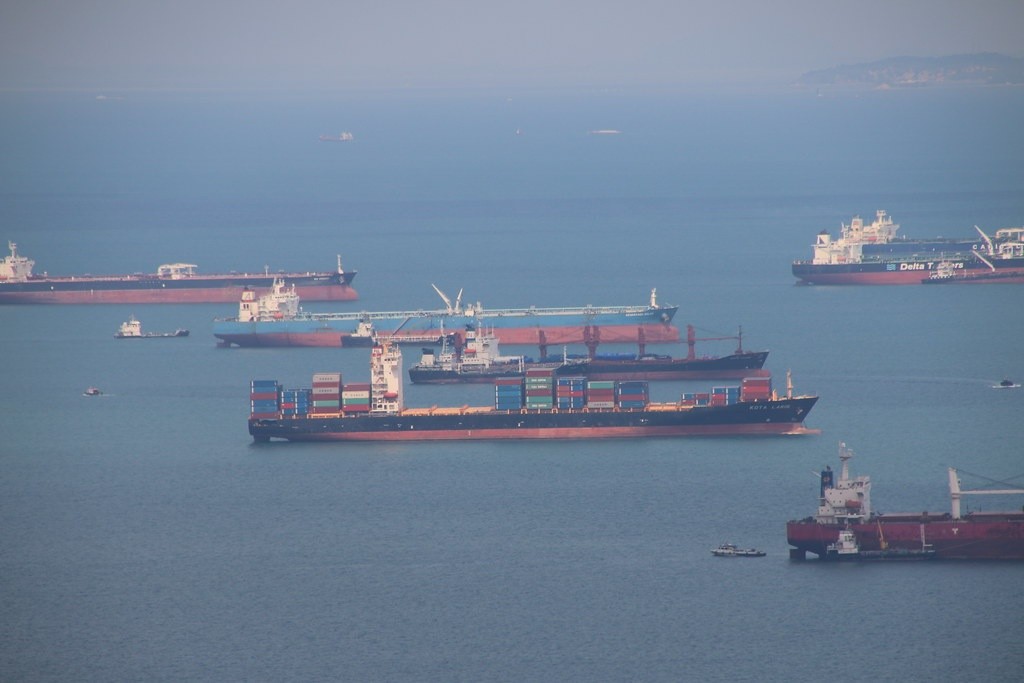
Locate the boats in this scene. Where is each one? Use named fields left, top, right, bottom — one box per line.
left=785, top=444, right=1023, bottom=559
left=406, top=317, right=769, bottom=383
left=0, top=240, right=360, bottom=302
left=113, top=314, right=189, bottom=337
left=83, top=386, right=103, bottom=395
left=211, top=275, right=678, bottom=344
left=789, top=209, right=1024, bottom=285
left=249, top=346, right=820, bottom=435
left=711, top=540, right=762, bottom=557
